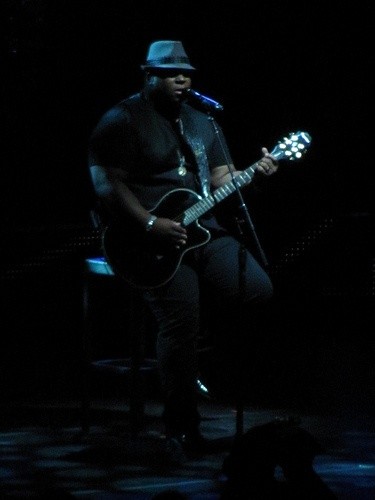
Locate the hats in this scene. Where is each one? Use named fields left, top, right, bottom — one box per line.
left=140, top=41, right=196, bottom=70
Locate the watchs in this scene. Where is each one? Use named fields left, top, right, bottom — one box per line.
left=145, top=215, right=157, bottom=233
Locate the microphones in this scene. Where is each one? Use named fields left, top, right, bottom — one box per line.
left=185, top=89, right=223, bottom=109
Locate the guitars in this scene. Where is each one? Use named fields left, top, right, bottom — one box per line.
left=100, top=130, right=313, bottom=290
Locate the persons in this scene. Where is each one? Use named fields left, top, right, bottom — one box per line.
left=95, top=39, right=275, bottom=442
